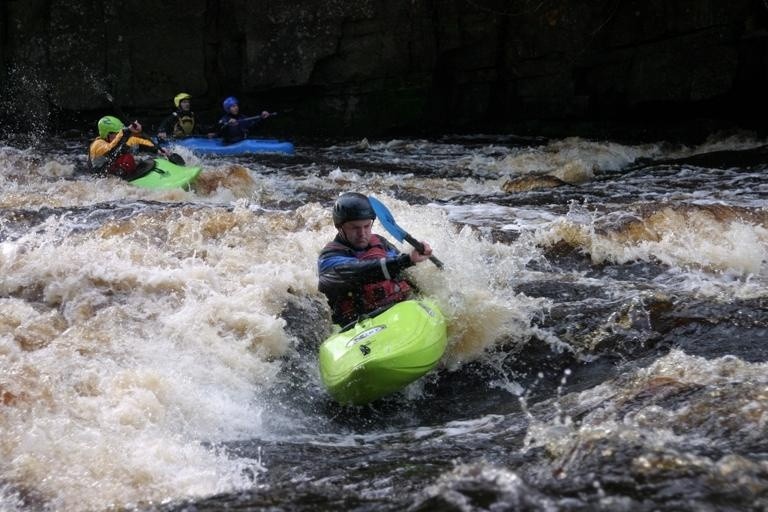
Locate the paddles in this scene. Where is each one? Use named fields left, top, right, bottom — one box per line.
left=208, top=107, right=294, bottom=129
left=369, top=198, right=445, bottom=271
left=131, top=124, right=186, bottom=166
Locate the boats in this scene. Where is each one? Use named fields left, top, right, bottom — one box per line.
left=150, top=134, right=298, bottom=160
left=317, top=288, right=448, bottom=408
left=126, top=154, right=201, bottom=191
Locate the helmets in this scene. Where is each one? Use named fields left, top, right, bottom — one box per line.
left=332, top=192, right=376, bottom=227
left=174, top=93, right=193, bottom=108
left=222, top=97, right=238, bottom=112
left=98, top=116, right=126, bottom=142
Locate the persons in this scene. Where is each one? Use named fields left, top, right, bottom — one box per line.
left=216, top=97, right=271, bottom=146
left=316, top=192, right=435, bottom=328
left=86, top=115, right=169, bottom=177
left=158, top=93, right=217, bottom=137
